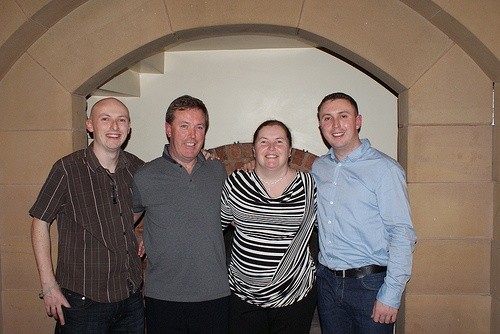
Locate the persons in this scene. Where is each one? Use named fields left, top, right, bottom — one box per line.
left=138, top=119, right=318, bottom=334
left=131, top=94, right=235, bottom=334
left=243, top=91, right=416, bottom=334
left=29, top=96, right=221, bottom=333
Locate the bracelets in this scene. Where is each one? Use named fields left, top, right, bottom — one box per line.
left=38, top=282, right=58, bottom=298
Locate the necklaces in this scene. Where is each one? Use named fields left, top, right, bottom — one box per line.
left=258, top=176, right=283, bottom=191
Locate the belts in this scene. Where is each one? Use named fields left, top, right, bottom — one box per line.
left=329, top=264, right=386, bottom=279
